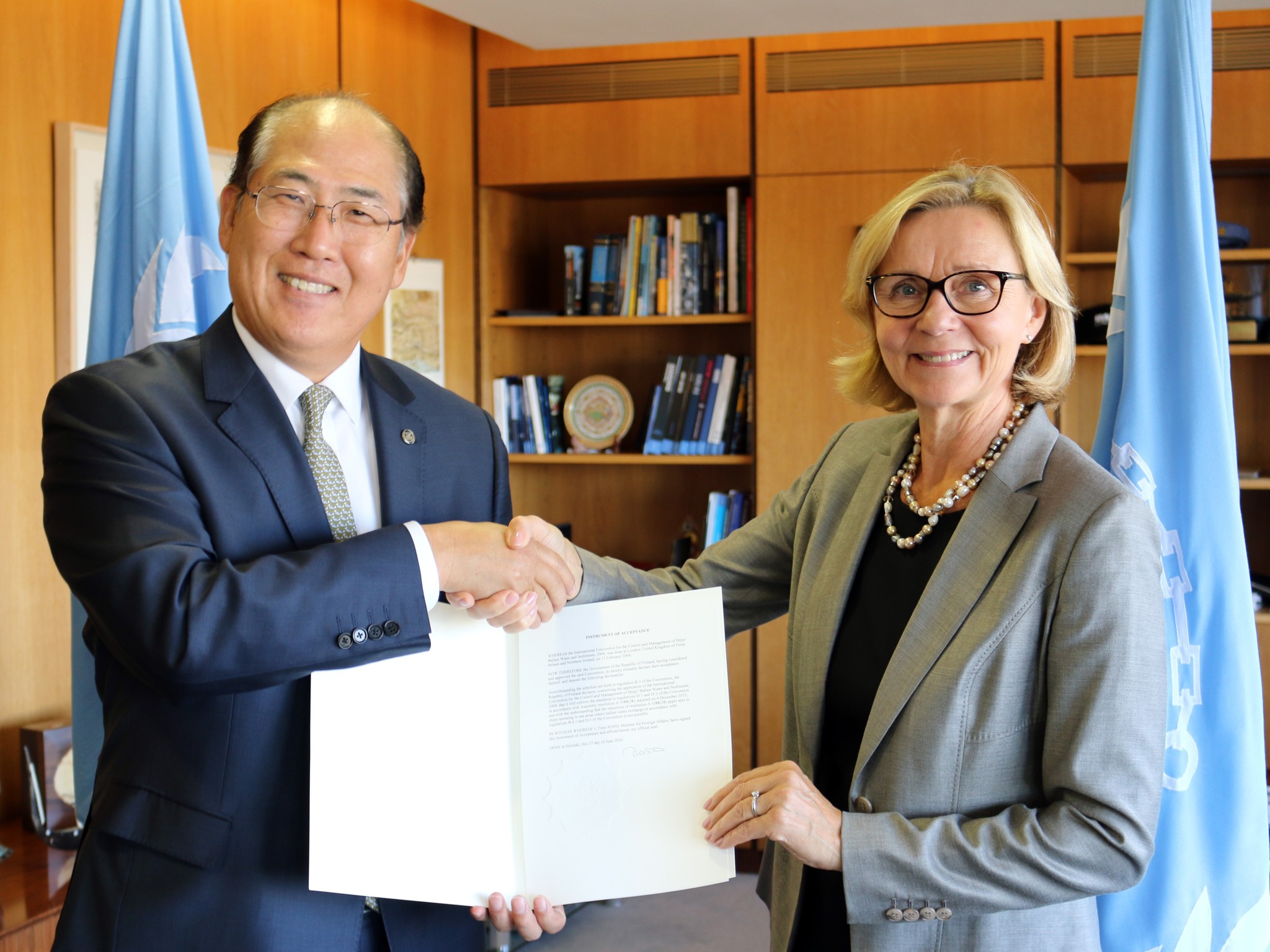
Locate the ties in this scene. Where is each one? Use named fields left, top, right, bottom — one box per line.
left=299, top=386, right=359, bottom=542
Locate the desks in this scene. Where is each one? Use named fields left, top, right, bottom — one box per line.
left=0, top=821, right=77, bottom=952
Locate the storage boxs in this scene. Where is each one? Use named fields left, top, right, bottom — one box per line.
left=20, top=721, right=76, bottom=831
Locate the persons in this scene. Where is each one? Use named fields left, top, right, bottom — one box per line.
left=443, top=164, right=1168, bottom=952
left=40, top=95, right=571, bottom=952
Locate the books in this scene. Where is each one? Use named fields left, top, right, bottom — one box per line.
left=562, top=185, right=748, bottom=315
left=490, top=373, right=566, bottom=456
left=643, top=351, right=754, bottom=456
left=1250, top=576, right=1270, bottom=594
left=1238, top=462, right=1270, bottom=477
left=1226, top=315, right=1270, bottom=344
left=703, top=490, right=752, bottom=550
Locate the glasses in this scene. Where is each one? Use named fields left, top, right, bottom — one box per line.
left=867, top=270, right=1031, bottom=319
left=246, top=185, right=402, bottom=246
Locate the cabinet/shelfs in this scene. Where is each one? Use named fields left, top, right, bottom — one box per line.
left=477, top=10, right=1270, bottom=773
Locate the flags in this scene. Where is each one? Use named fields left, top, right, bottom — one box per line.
left=1092, top=0, right=1270, bottom=952
left=70, top=0, right=234, bottom=831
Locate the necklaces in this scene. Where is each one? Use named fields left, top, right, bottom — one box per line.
left=883, top=399, right=1030, bottom=549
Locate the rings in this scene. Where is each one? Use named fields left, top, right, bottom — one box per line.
left=750, top=791, right=759, bottom=818
